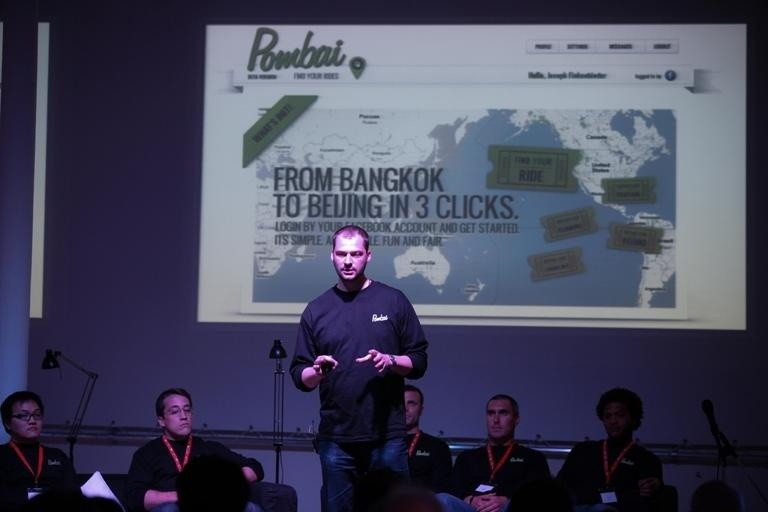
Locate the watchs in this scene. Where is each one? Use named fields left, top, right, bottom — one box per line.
left=388, top=354, right=396, bottom=370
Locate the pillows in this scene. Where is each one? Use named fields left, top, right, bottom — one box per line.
left=77, top=471, right=125, bottom=512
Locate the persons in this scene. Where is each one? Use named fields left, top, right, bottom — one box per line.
left=289, top=226, right=429, bottom=512
left=555, top=386, right=663, bottom=511
left=0, top=388, right=264, bottom=512
left=404, top=385, right=554, bottom=511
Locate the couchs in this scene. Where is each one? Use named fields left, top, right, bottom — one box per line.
left=1, top=471, right=297, bottom=511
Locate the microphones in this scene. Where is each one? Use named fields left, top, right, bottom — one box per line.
left=701, top=398, right=727, bottom=468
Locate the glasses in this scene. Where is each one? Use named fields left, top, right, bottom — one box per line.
left=11, top=413, right=41, bottom=420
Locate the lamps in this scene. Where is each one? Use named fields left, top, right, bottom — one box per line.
left=38, top=349, right=100, bottom=466
left=268, top=338, right=288, bottom=481
